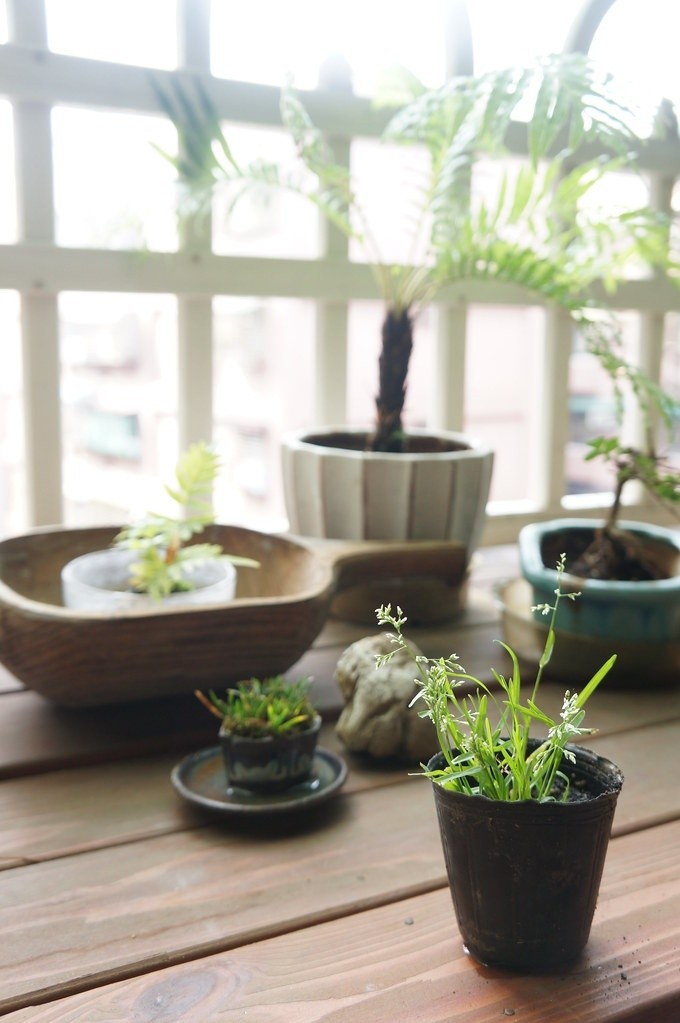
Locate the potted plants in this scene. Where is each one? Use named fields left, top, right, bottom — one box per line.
left=200, top=675, right=322, bottom=790
left=517, top=437, right=680, bottom=675
left=151, top=43, right=680, bottom=627
left=48, top=442, right=251, bottom=613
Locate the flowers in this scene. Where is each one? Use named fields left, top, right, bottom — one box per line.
left=373, top=551, right=618, bottom=791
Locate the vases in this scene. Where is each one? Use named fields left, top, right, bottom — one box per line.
left=428, top=739, right=624, bottom=971
